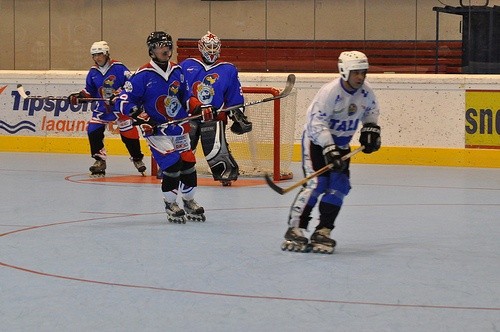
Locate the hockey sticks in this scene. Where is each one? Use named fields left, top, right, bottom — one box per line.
left=150, top=73, right=296, bottom=129
left=264, top=145, right=366, bottom=195
left=16, top=84, right=113, bottom=102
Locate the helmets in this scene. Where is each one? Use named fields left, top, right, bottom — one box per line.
left=198, top=31, right=222, bottom=63
left=146, top=31, right=172, bottom=49
left=91, top=41, right=109, bottom=57
left=339, top=50, right=368, bottom=81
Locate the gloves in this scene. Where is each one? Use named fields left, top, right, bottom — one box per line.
left=128, top=105, right=158, bottom=137
left=68, top=89, right=91, bottom=105
left=104, top=93, right=120, bottom=106
left=192, top=103, right=217, bottom=123
left=322, top=145, right=346, bottom=172
left=359, top=123, right=381, bottom=154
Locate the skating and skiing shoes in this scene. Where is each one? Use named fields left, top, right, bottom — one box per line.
left=221, top=180, right=232, bottom=186
left=181, top=196, right=206, bottom=222
left=88, top=155, right=107, bottom=177
left=310, top=227, right=336, bottom=253
left=163, top=196, right=186, bottom=224
left=130, top=157, right=147, bottom=176
left=281, top=227, right=309, bottom=252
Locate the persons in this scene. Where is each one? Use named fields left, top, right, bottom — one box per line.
left=178, top=30, right=252, bottom=188
left=115, top=32, right=216, bottom=223
left=67, top=41, right=147, bottom=178
left=281, top=50, right=382, bottom=255
